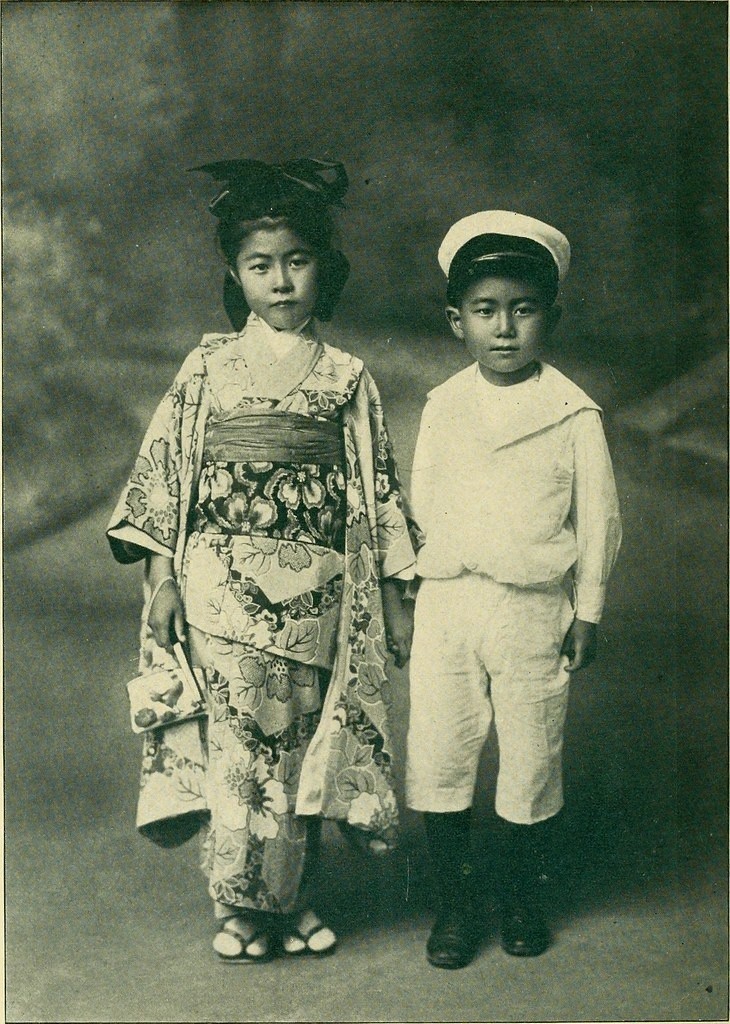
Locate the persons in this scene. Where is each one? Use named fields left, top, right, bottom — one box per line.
left=404, top=209, right=624, bottom=971
left=103, top=155, right=426, bottom=962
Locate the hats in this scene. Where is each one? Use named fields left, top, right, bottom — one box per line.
left=438, top=210, right=572, bottom=307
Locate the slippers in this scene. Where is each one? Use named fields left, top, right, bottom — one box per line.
left=214, top=922, right=337, bottom=963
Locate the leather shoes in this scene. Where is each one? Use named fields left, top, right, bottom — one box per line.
left=428, top=878, right=551, bottom=969
